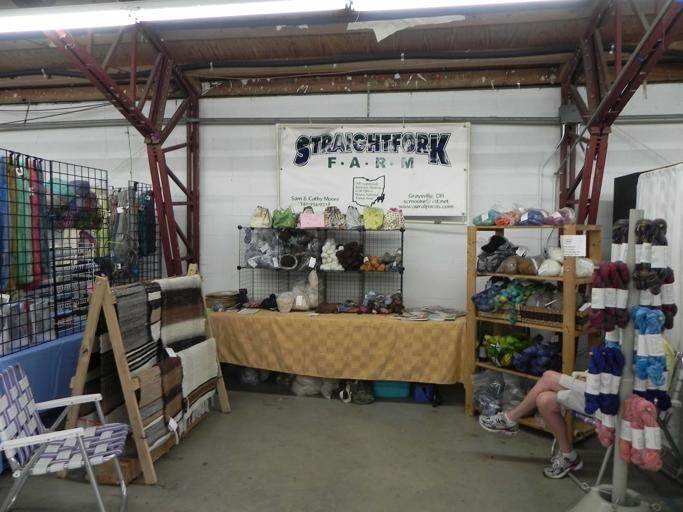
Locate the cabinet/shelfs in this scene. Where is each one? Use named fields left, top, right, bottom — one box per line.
left=237, top=222, right=405, bottom=311
left=112, top=180, right=164, bottom=283
left=56, top=275, right=232, bottom=484
left=466, top=219, right=602, bottom=439
left=3, top=150, right=109, bottom=357
left=572, top=208, right=675, bottom=511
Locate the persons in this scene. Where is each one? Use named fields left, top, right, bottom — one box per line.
left=479, top=335, right=676, bottom=479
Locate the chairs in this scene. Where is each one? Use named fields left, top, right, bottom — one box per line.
left=1, top=363, right=129, bottom=512
left=549, top=355, right=681, bottom=494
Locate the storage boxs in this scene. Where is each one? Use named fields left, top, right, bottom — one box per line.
left=373, top=381, right=409, bottom=401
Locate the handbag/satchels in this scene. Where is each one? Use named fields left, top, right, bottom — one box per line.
left=296, top=206, right=324, bottom=230
left=324, top=206, right=346, bottom=231
left=346, top=205, right=363, bottom=231
left=271, top=204, right=296, bottom=230
left=249, top=205, right=272, bottom=228
left=385, top=209, right=406, bottom=230
left=363, top=206, right=385, bottom=231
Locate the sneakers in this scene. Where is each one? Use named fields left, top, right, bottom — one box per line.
left=478, top=411, right=520, bottom=437
left=542, top=450, right=584, bottom=480
